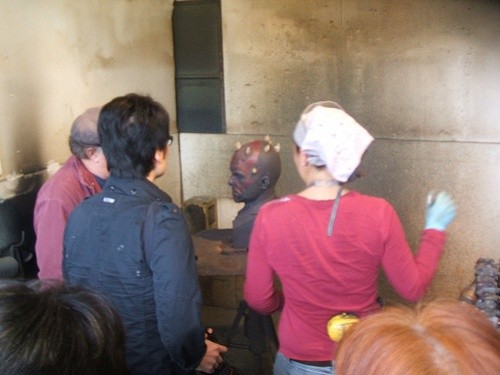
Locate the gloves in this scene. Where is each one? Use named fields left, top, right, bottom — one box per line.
left=425, top=190, right=457, bottom=232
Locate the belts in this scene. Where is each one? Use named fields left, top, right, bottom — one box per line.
left=290, top=359, right=333, bottom=366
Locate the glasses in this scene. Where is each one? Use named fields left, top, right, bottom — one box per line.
left=152, top=136, right=173, bottom=158
left=301, top=101, right=344, bottom=115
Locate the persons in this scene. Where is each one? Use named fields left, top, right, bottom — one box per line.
left=226, top=132, right=283, bottom=250
left=240, top=101, right=457, bottom=375
left=0, top=277, right=129, bottom=375
left=331, top=297, right=500, bottom=375
left=33, top=105, right=113, bottom=296
left=62, top=93, right=228, bottom=375
